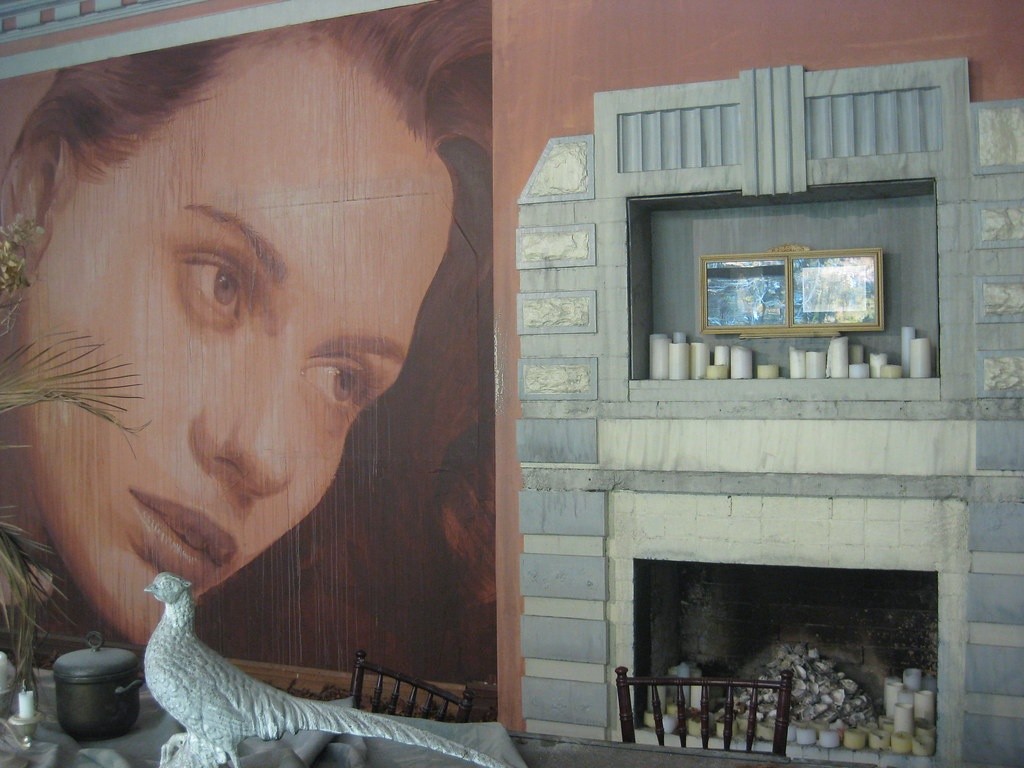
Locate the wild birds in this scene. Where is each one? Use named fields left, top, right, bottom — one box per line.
left=141, top=572, right=530, bottom=768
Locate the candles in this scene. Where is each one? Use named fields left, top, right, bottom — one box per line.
left=18, top=688, right=35, bottom=719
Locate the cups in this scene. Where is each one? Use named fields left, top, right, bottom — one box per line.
left=885, top=668, right=937, bottom=735
left=648, top=323, right=932, bottom=381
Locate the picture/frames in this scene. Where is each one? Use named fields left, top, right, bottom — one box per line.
left=698, top=247, right=885, bottom=337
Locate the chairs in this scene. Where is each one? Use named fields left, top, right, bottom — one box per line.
left=349, top=650, right=476, bottom=724
left=614, top=665, right=792, bottom=763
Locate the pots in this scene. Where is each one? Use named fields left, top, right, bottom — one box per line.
left=0, top=651, right=18, bottom=723
left=53, top=631, right=144, bottom=742
